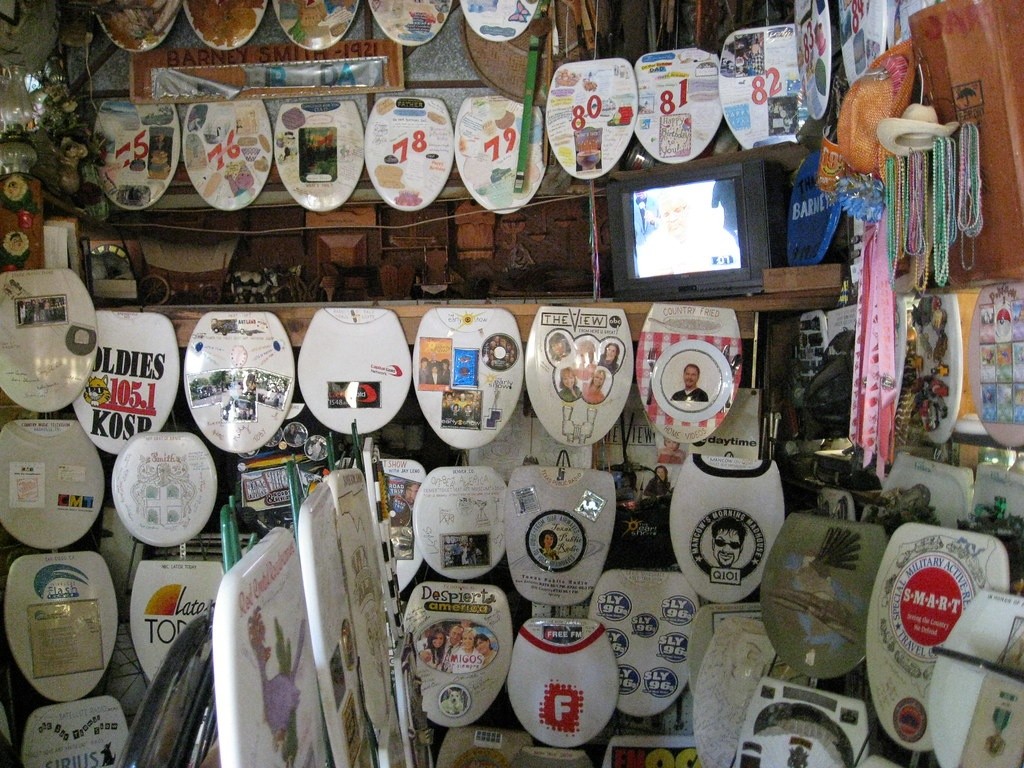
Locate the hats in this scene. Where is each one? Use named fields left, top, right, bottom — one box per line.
left=837, top=38, right=915, bottom=191
left=876, top=103, right=959, bottom=156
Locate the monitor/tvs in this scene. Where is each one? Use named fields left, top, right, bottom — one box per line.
left=606, top=159, right=788, bottom=301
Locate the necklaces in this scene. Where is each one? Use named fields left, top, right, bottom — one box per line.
left=883, top=120, right=984, bottom=296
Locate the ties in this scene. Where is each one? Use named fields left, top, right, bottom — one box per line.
left=441, top=645, right=453, bottom=672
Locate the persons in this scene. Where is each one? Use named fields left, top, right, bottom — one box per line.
left=546, top=331, right=621, bottom=405
left=669, top=364, right=708, bottom=402
left=473, top=633, right=497, bottom=669
left=448, top=628, right=484, bottom=674
left=195, top=334, right=519, bottom=509
left=418, top=627, right=446, bottom=669
left=642, top=465, right=670, bottom=497
left=449, top=533, right=483, bottom=566
left=435, top=623, right=464, bottom=671
left=538, top=529, right=560, bottom=560
left=656, top=435, right=686, bottom=463
